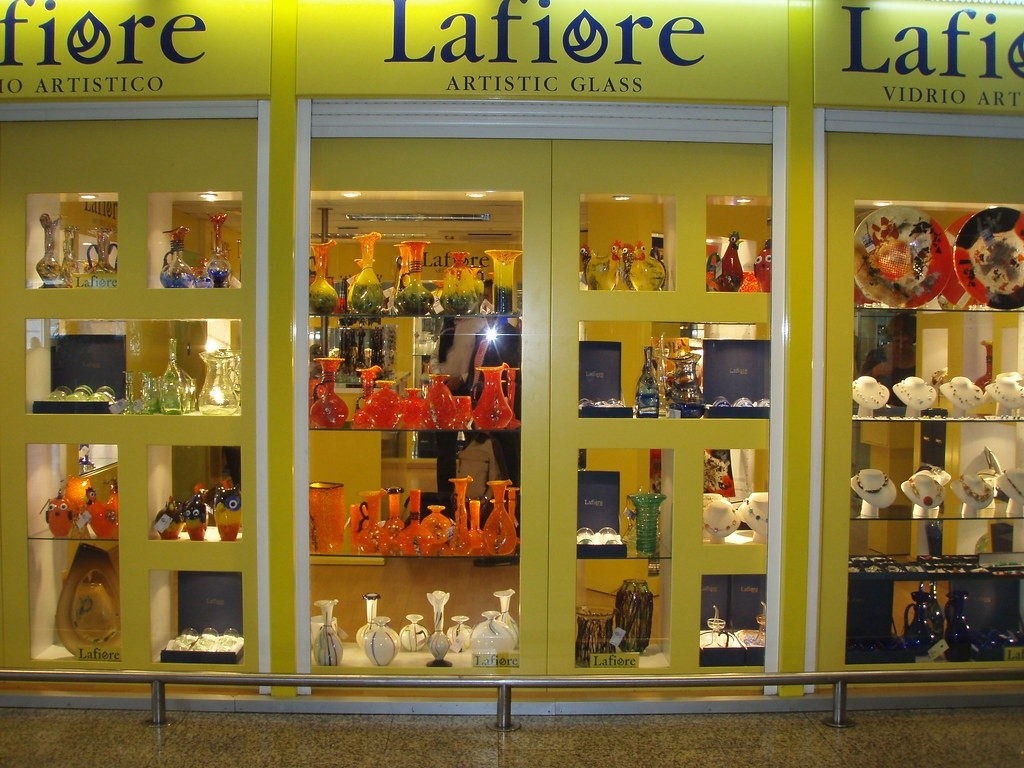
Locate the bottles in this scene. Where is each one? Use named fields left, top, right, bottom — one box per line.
left=576, top=579, right=667, bottom=667
left=307, top=476, right=527, bottom=556
left=161, top=339, right=182, bottom=415
left=48, top=384, right=112, bottom=402
left=896, top=580, right=1024, bottom=663
left=636, top=348, right=659, bottom=415
left=309, top=230, right=530, bottom=315
left=307, top=358, right=520, bottom=432
left=157, top=209, right=237, bottom=288
left=35, top=213, right=123, bottom=289
left=309, top=586, right=518, bottom=670
left=577, top=527, right=620, bottom=545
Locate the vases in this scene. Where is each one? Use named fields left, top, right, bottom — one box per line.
left=36, top=208, right=229, bottom=288
left=309, top=232, right=521, bottom=666
left=38, top=474, right=242, bottom=542
left=577, top=227, right=772, bottom=664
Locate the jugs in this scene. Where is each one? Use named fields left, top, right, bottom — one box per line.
left=199, top=349, right=240, bottom=415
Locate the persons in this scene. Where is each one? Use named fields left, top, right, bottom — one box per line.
left=420, top=278, right=521, bottom=530
left=861, top=312, right=916, bottom=406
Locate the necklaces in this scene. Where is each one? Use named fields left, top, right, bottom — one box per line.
left=899, top=380, right=933, bottom=403
left=984, top=450, right=993, bottom=470
left=1004, top=469, right=1023, bottom=500
left=959, top=475, right=992, bottom=502
left=992, top=383, right=1024, bottom=403
left=745, top=499, right=769, bottom=525
left=949, top=383, right=981, bottom=404
left=909, top=478, right=942, bottom=507
left=853, top=382, right=886, bottom=404
left=857, top=470, right=889, bottom=494
left=703, top=509, right=737, bottom=534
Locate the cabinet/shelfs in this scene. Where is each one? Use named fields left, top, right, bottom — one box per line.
left=0, top=114, right=256, bottom=671
left=545, top=137, right=774, bottom=675
left=812, top=130, right=1024, bottom=672
left=308, top=135, right=555, bottom=679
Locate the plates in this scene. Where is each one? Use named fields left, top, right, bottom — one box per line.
left=855, top=205, right=1024, bottom=309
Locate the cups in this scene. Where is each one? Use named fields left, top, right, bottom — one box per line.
left=125, top=370, right=152, bottom=414
